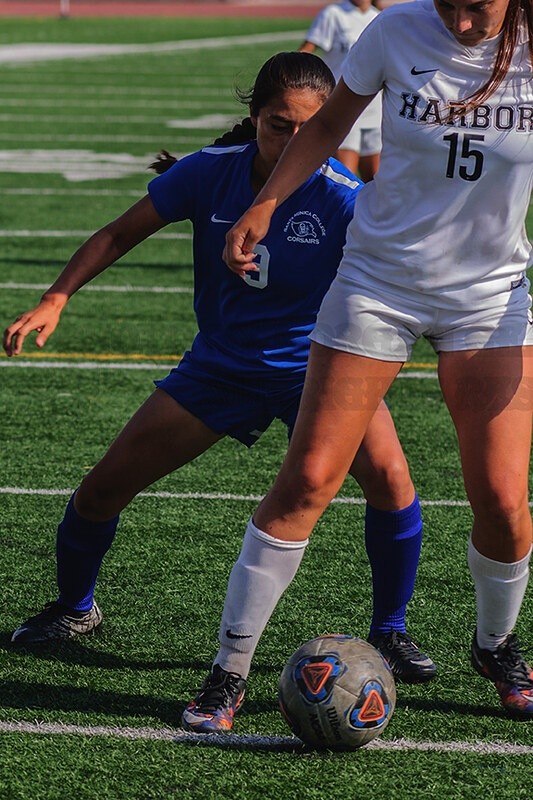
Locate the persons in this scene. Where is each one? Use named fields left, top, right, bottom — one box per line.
left=181, top=0, right=533, bottom=732
left=1, top=52, right=436, bottom=678
left=299, top=0, right=393, bottom=192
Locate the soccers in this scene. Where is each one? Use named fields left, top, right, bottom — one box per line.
left=277, top=635, right=396, bottom=751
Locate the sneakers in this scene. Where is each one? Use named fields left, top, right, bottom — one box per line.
left=13, top=597, right=102, bottom=643
left=471, top=628, right=533, bottom=719
left=366, top=630, right=437, bottom=682
left=182, top=662, right=248, bottom=732
